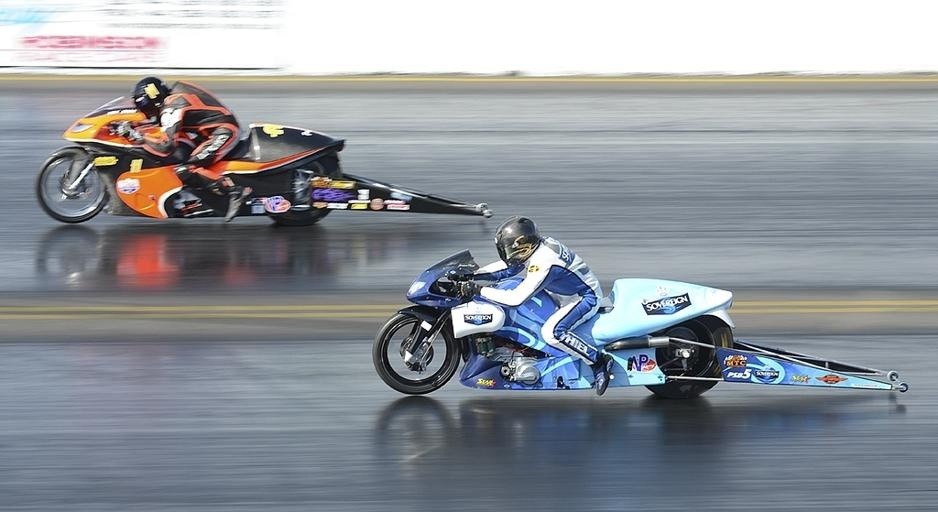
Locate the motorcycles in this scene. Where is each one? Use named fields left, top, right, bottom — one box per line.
left=371, top=247, right=736, bottom=400
left=35, top=95, right=346, bottom=226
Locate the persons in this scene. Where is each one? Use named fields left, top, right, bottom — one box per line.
left=447, top=214, right=616, bottom=398
left=107, top=77, right=250, bottom=225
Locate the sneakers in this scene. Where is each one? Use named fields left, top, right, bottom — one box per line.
left=587, top=349, right=615, bottom=396
left=224, top=185, right=253, bottom=222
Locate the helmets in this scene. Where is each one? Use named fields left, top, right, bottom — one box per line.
left=493, top=213, right=541, bottom=270
left=132, top=77, right=170, bottom=118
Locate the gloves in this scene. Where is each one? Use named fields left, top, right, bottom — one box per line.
left=456, top=279, right=483, bottom=301
